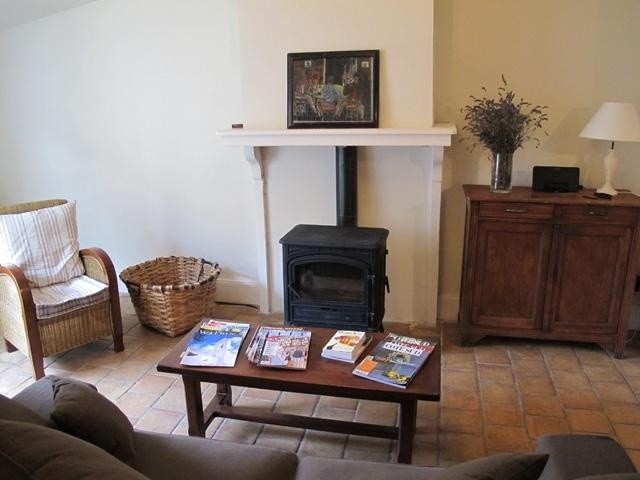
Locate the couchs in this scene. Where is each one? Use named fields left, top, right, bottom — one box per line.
left=0, top=374, right=639, bottom=480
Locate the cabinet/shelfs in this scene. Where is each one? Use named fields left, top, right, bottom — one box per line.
left=458, top=184, right=640, bottom=359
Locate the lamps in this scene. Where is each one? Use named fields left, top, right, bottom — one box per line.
left=577, top=101, right=640, bottom=196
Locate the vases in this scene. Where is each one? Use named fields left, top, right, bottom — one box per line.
left=490, top=152, right=515, bottom=194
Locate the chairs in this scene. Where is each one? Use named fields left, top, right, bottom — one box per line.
left=0, top=198, right=125, bottom=381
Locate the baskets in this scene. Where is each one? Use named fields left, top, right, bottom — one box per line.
left=119, top=254, right=223, bottom=338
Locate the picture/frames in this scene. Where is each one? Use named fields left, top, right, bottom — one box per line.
left=285, top=49, right=380, bottom=129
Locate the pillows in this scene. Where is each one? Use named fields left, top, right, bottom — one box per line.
left=0, top=199, right=85, bottom=290
left=49, top=374, right=136, bottom=465
left=430, top=453, right=550, bottom=480
left=1, top=419, right=151, bottom=480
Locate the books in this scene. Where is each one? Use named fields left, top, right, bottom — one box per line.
left=180, top=319, right=312, bottom=370
left=320, top=329, right=373, bottom=364
left=352, top=332, right=438, bottom=390
